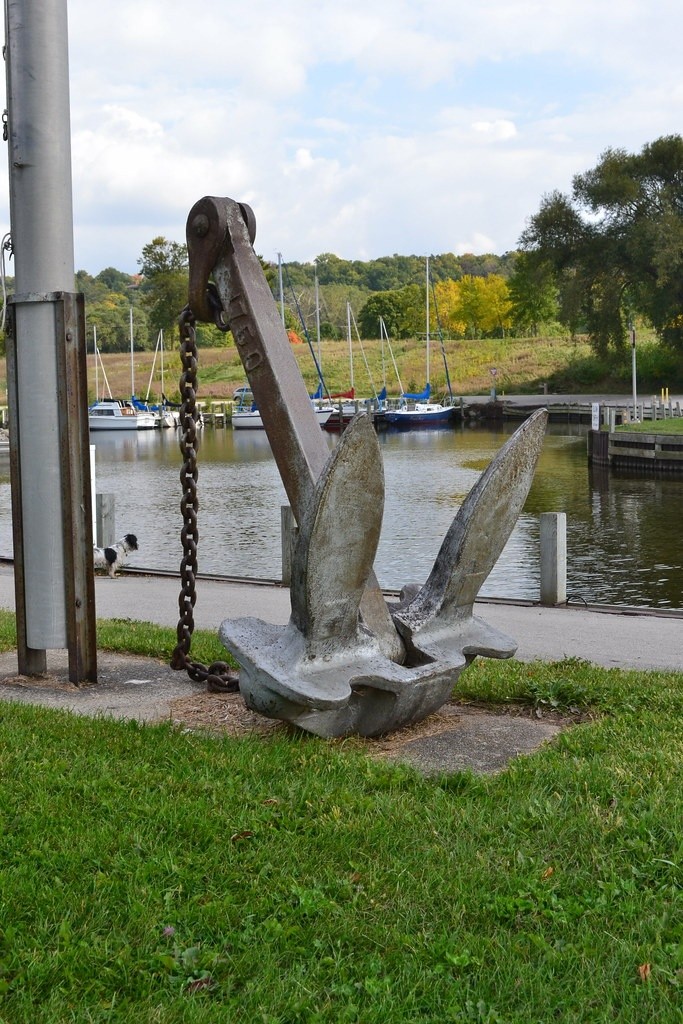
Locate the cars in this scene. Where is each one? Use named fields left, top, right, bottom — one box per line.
left=232, top=388, right=255, bottom=403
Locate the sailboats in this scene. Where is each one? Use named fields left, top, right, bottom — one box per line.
left=378, top=255, right=471, bottom=425
left=88, top=307, right=161, bottom=430
left=324, top=300, right=387, bottom=427
left=141, top=328, right=184, bottom=427
left=232, top=251, right=333, bottom=429
